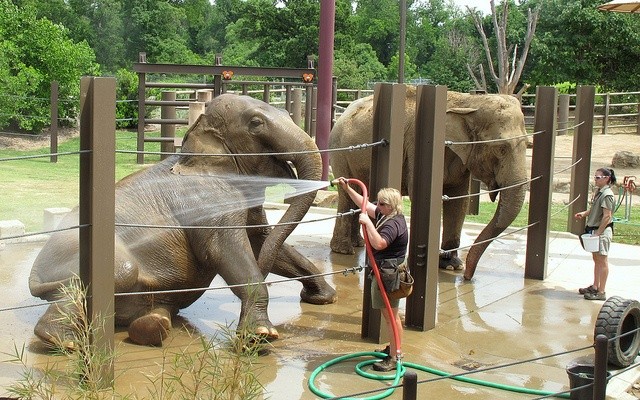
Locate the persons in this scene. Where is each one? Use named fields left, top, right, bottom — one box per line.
left=575, top=168, right=615, bottom=300
left=339, top=177, right=407, bottom=371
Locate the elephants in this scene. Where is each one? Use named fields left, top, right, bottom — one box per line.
left=327, top=83, right=530, bottom=281
left=28, top=92, right=339, bottom=353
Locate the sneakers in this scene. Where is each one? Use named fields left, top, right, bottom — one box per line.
left=375, top=344, right=390, bottom=359
left=584, top=290, right=607, bottom=300
left=578, top=284, right=600, bottom=294
left=373, top=353, right=401, bottom=372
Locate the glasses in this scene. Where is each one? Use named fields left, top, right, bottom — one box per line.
left=594, top=176, right=608, bottom=179
left=379, top=199, right=386, bottom=206
